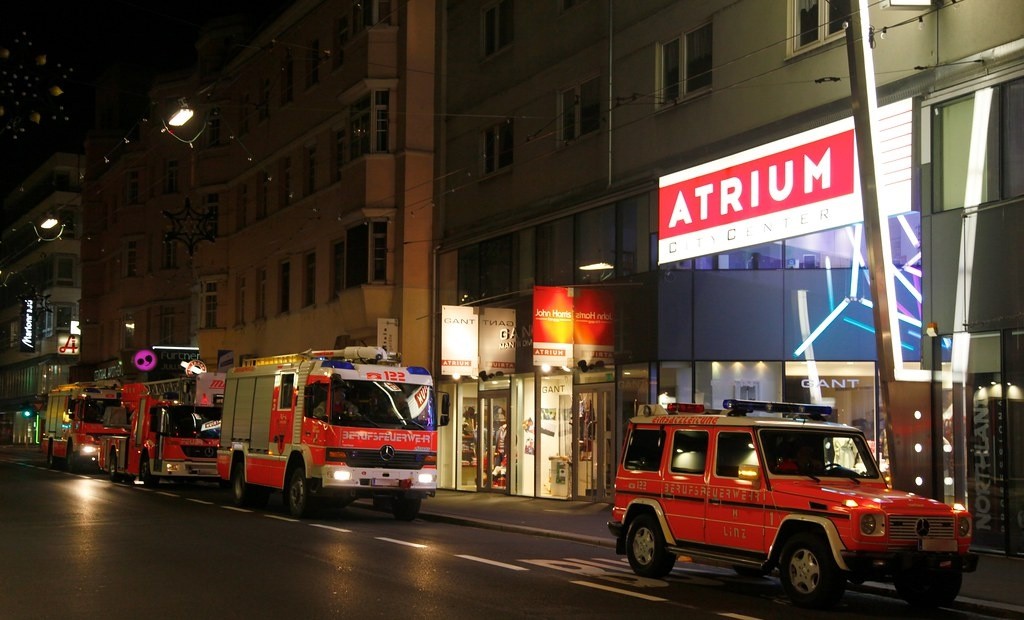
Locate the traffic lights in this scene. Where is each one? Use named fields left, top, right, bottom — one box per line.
left=23, top=411, right=32, bottom=418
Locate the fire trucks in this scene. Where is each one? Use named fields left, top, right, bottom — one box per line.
left=97, top=371, right=232, bottom=492
left=39, top=380, right=126, bottom=476
left=216, top=345, right=451, bottom=521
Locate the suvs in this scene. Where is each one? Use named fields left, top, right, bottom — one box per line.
left=607, top=397, right=980, bottom=605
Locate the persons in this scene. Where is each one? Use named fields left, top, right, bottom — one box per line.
left=494, top=408, right=506, bottom=421
left=313, top=388, right=358, bottom=419
left=495, top=424, right=508, bottom=463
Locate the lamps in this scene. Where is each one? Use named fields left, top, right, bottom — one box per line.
left=39, top=210, right=59, bottom=229
left=163, top=96, right=195, bottom=127
